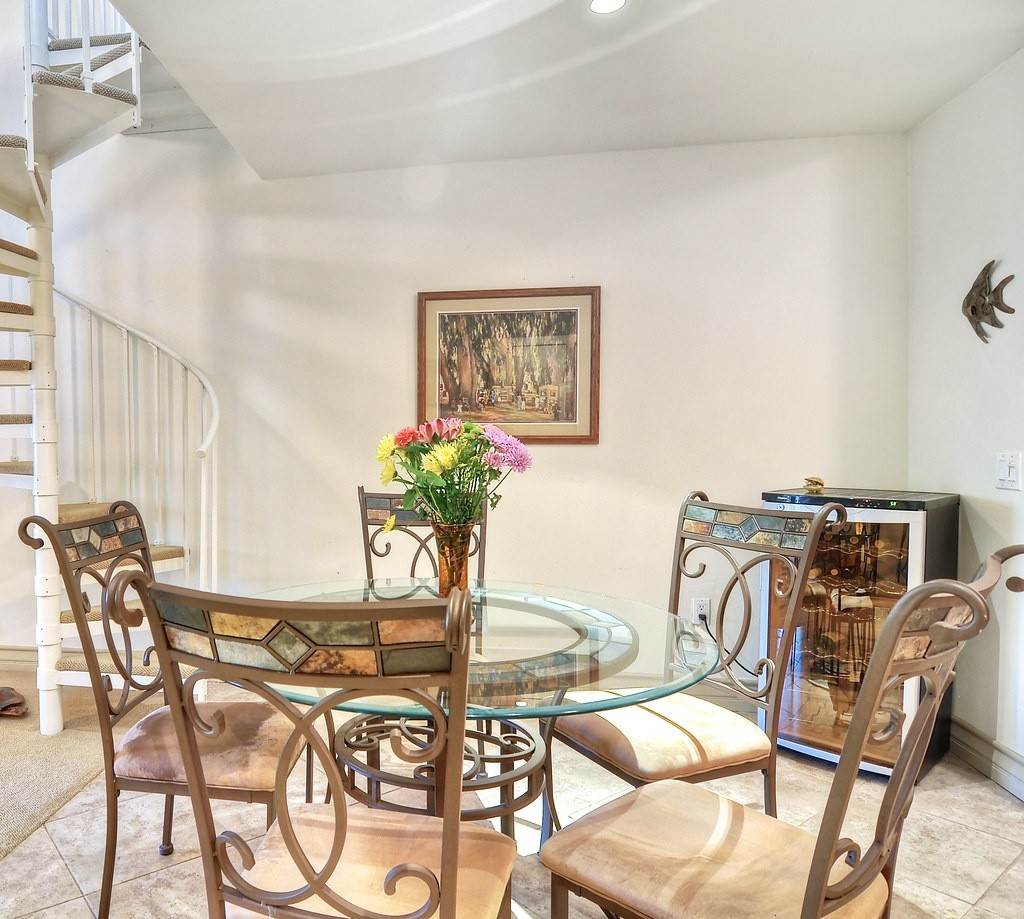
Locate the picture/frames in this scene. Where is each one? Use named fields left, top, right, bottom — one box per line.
left=417, top=286, right=602, bottom=444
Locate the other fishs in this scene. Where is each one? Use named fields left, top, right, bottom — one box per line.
left=960, top=259, right=1015, bottom=344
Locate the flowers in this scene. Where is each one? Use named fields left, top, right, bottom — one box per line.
left=375, top=414, right=532, bottom=595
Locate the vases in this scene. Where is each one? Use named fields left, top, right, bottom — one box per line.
left=430, top=523, right=475, bottom=598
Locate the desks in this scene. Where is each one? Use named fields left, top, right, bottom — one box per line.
left=217, top=578, right=721, bottom=919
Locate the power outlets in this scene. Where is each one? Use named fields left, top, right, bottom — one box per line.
left=692, top=598, right=711, bottom=626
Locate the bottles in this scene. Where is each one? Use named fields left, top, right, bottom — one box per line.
left=808, top=532, right=906, bottom=580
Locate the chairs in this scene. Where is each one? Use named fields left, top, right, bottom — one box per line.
left=537, top=544, right=1024, bottom=919
left=144, top=580, right=517, bottom=919
left=358, top=486, right=487, bottom=779
left=539, top=489, right=847, bottom=854
left=18, top=500, right=316, bottom=919
